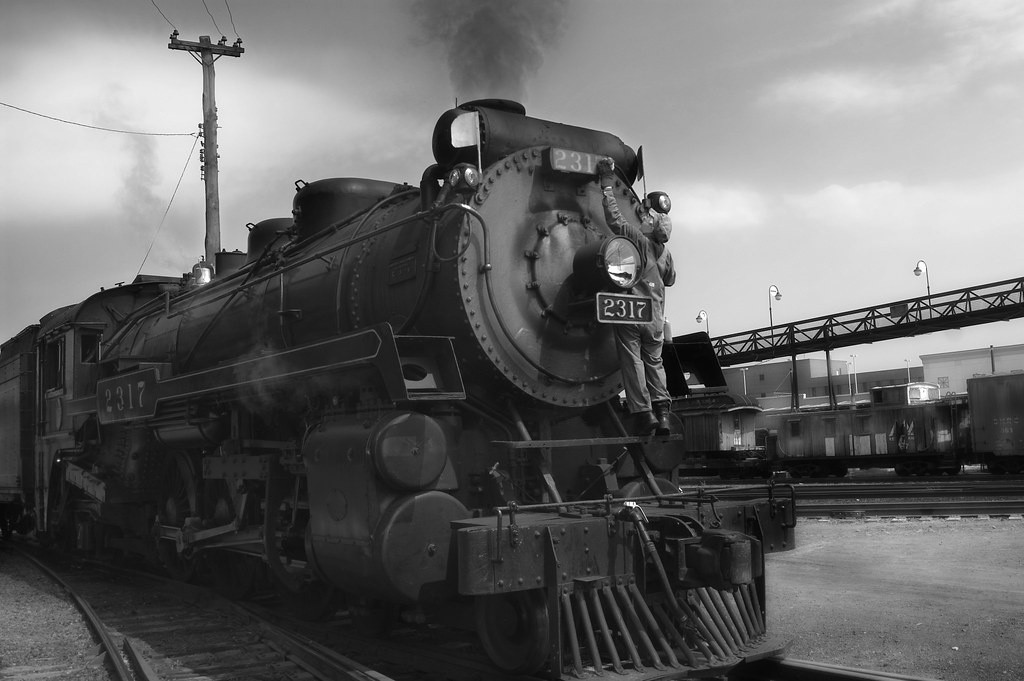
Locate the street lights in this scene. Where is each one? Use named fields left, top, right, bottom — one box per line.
left=914, top=260, right=932, bottom=320
left=696, top=310, right=709, bottom=335
left=769, top=284, right=782, bottom=347
left=740, top=368, right=748, bottom=395
left=904, top=359, right=911, bottom=383
left=850, top=354, right=858, bottom=394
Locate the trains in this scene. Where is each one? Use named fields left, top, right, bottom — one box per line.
left=659, top=332, right=796, bottom=558
left=0, top=96, right=802, bottom=681
left=670, top=370, right=1024, bottom=484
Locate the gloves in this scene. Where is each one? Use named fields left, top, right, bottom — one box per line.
left=597, top=159, right=616, bottom=189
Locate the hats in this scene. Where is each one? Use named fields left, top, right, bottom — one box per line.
left=649, top=207, right=672, bottom=242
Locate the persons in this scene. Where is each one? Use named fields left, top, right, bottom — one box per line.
left=597, top=157, right=676, bottom=437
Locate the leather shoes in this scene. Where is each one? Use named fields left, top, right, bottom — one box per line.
left=655, top=402, right=671, bottom=435
left=634, top=412, right=659, bottom=436
